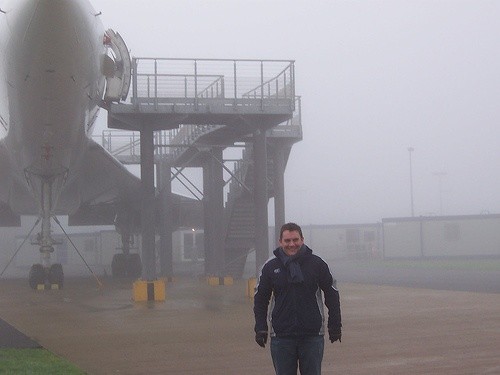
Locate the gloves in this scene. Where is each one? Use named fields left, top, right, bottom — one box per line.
left=255, top=329, right=268, bottom=348
left=328, top=324, right=342, bottom=344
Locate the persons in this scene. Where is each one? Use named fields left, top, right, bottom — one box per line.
left=254, top=222, right=342, bottom=375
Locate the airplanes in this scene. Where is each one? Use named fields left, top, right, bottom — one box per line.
left=0, top=0, right=202, bottom=289
